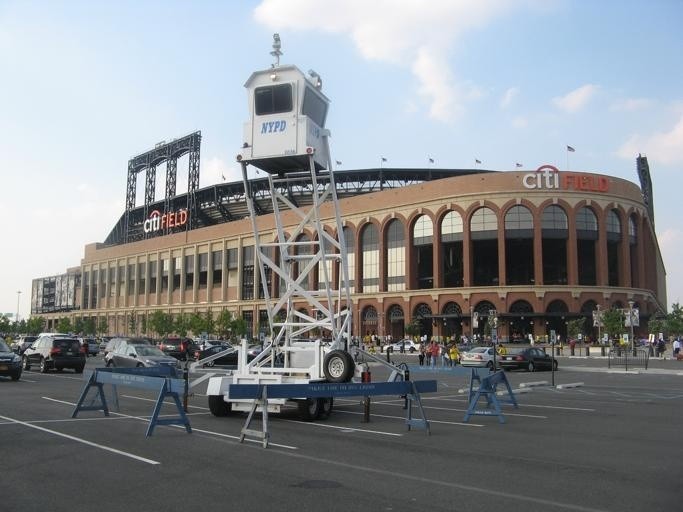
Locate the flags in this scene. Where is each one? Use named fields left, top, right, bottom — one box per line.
left=567, top=145, right=575, bottom=153
left=475, top=159, right=481, bottom=164
left=429, top=158, right=434, bottom=163
left=336, top=161, right=342, bottom=165
left=514, top=163, right=523, bottom=168
left=382, top=157, right=388, bottom=162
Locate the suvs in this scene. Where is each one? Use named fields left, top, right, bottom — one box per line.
left=9, top=335, right=39, bottom=356
left=22, top=332, right=87, bottom=374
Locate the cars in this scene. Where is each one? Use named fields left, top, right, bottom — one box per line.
left=78, top=335, right=264, bottom=366
left=499, top=347, right=559, bottom=373
left=106, top=344, right=181, bottom=377
left=383, top=339, right=421, bottom=353
left=566, top=336, right=662, bottom=348
left=0, top=337, right=23, bottom=381
left=459, top=346, right=502, bottom=371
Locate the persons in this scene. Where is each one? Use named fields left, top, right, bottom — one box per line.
left=94, top=336, right=102, bottom=355
left=568, top=336, right=576, bottom=356
left=362, top=329, right=508, bottom=372
left=607, top=333, right=666, bottom=359
left=81, top=338, right=91, bottom=358
left=672, top=338, right=680, bottom=355
left=4, top=334, right=11, bottom=345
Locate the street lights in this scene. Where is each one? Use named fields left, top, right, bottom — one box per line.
left=628, top=298, right=636, bottom=360
left=468, top=303, right=475, bottom=343
left=595, top=303, right=601, bottom=347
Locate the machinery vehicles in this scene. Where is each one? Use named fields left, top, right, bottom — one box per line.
left=200, top=23, right=357, bottom=421
